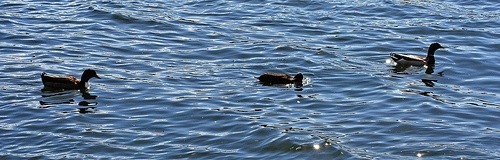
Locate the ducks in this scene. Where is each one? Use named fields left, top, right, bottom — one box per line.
left=256, top=72, right=304, bottom=84
left=41, top=69, right=101, bottom=92
left=390, top=42, right=444, bottom=66
